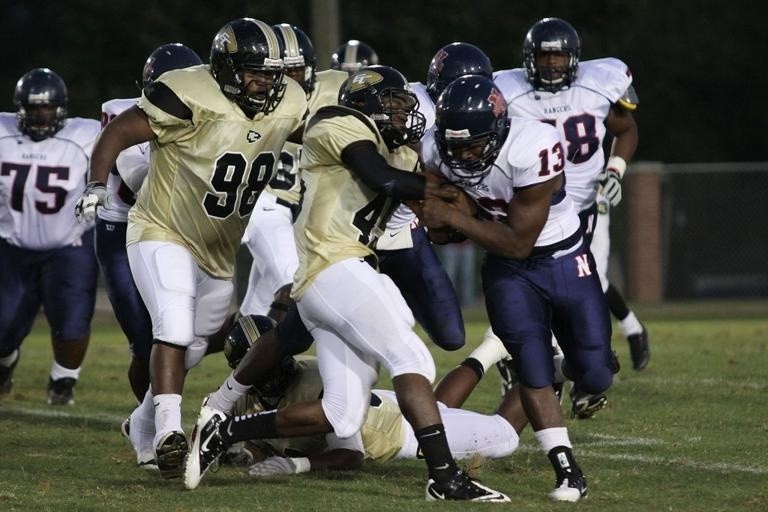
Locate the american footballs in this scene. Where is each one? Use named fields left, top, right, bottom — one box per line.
left=406, top=172, right=442, bottom=213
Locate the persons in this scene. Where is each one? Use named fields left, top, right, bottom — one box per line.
left=0, top=67, right=102, bottom=406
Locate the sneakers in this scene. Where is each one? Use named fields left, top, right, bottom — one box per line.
left=0, top=346, right=19, bottom=395
left=46, top=360, right=80, bottom=405
left=571, top=393, right=607, bottom=421
left=548, top=468, right=588, bottom=502
left=628, top=321, right=650, bottom=371
left=424, top=475, right=512, bottom=503
left=496, top=350, right=565, bottom=406
left=181, top=395, right=233, bottom=491
left=122, top=411, right=187, bottom=481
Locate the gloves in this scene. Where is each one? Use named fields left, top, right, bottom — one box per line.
left=74, top=182, right=112, bottom=222
left=596, top=167, right=622, bottom=207
left=247, top=452, right=294, bottom=478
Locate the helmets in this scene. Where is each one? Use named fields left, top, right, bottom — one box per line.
left=210, top=17, right=287, bottom=114
left=522, top=18, right=580, bottom=94
left=337, top=65, right=426, bottom=152
left=434, top=76, right=508, bottom=179
left=426, top=42, right=494, bottom=102
left=274, top=22, right=316, bottom=96
left=328, top=38, right=378, bottom=72
left=141, top=43, right=203, bottom=92
left=224, top=313, right=295, bottom=399
left=13, top=69, right=68, bottom=141
